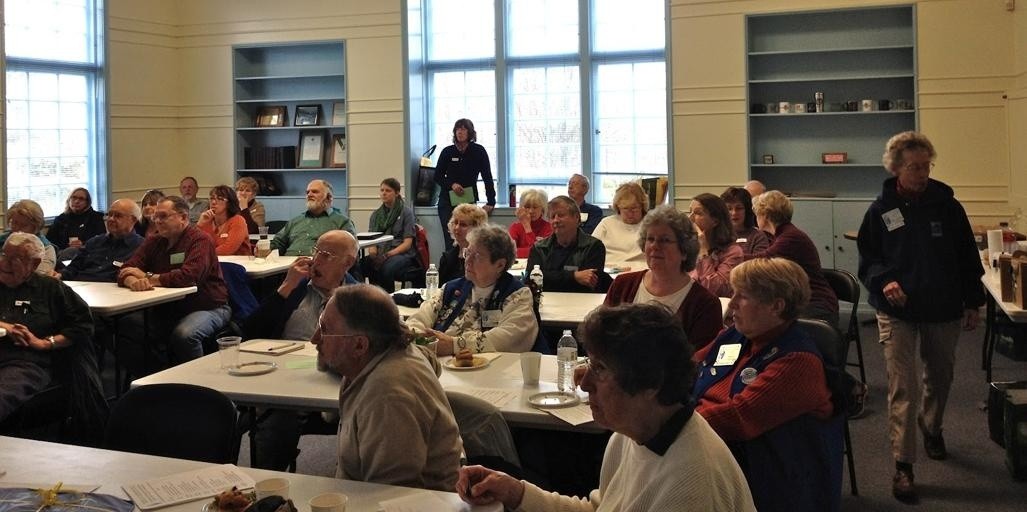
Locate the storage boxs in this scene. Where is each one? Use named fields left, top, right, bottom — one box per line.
left=243, top=146, right=296, bottom=170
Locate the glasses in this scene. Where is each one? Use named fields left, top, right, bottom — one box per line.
left=310, top=246, right=336, bottom=262
left=903, top=161, right=935, bottom=172
left=585, top=357, right=612, bottom=382
left=463, top=248, right=488, bottom=261
left=318, top=314, right=353, bottom=339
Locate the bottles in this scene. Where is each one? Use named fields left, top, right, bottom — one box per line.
left=529, top=265, right=544, bottom=313
left=995, top=221, right=1016, bottom=254
left=256, top=235, right=271, bottom=264
left=426, top=263, right=439, bottom=300
left=557, top=330, right=578, bottom=391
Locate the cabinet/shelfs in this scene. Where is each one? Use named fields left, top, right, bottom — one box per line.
left=231, top=37, right=350, bottom=251
left=745, top=4, right=918, bottom=316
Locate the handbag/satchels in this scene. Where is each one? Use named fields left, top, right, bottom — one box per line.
left=414, top=145, right=441, bottom=206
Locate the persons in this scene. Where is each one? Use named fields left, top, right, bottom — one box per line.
left=857, top=133, right=986, bottom=506
left=311, top=174, right=839, bottom=511
left=1, top=172, right=357, bottom=443
left=431, top=118, right=497, bottom=252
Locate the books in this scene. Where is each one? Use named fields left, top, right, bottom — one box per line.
left=242, top=145, right=298, bottom=169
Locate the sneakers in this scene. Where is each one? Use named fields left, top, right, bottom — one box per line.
left=892, top=471, right=917, bottom=499
left=847, top=381, right=868, bottom=419
left=918, top=414, right=945, bottom=459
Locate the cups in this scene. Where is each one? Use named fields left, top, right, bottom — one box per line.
left=879, top=99, right=895, bottom=110
left=308, top=494, right=349, bottom=512
left=814, top=90, right=825, bottom=113
left=258, top=226, right=269, bottom=236
left=519, top=352, right=542, bottom=385
left=846, top=100, right=859, bottom=111
left=862, top=99, right=873, bottom=111
left=217, top=336, right=242, bottom=368
left=69, top=237, right=78, bottom=247
left=752, top=101, right=816, bottom=113
left=254, top=477, right=291, bottom=502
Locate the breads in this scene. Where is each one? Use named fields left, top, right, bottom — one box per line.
left=213, top=487, right=247, bottom=511
left=454, top=350, right=474, bottom=366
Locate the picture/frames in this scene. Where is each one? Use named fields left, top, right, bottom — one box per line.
left=294, top=103, right=322, bottom=126
left=332, top=101, right=345, bottom=126
left=255, top=105, right=287, bottom=127
left=296, top=128, right=324, bottom=168
left=330, top=133, right=346, bottom=168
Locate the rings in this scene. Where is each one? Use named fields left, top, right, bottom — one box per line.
left=887, top=295, right=892, bottom=301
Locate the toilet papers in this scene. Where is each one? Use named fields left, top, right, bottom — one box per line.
left=987, top=229, right=1003, bottom=268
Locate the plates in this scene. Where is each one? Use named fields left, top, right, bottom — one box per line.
left=527, top=392, right=579, bottom=407
left=230, top=362, right=278, bottom=375
left=443, top=357, right=491, bottom=370
